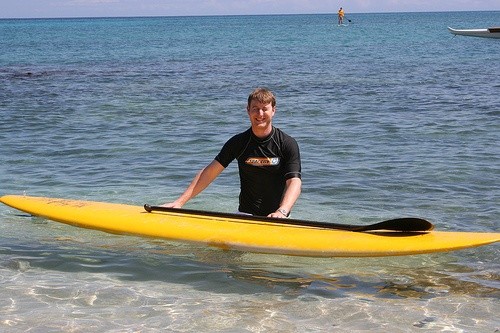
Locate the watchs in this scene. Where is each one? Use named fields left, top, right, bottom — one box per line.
left=279, top=209, right=287, bottom=216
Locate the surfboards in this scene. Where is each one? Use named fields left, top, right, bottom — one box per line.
left=337, top=24, right=348, bottom=27
left=0, top=194, right=500, bottom=257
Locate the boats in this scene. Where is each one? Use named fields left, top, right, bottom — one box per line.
left=337, top=23, right=349, bottom=28
left=0, top=194, right=500, bottom=257
left=447, top=26, right=500, bottom=40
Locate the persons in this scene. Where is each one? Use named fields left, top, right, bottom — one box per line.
left=338, top=8, right=344, bottom=24
left=158, top=87, right=302, bottom=219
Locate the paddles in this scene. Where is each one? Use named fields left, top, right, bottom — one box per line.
left=343, top=16, right=351, bottom=22
left=144, top=204, right=433, bottom=232
